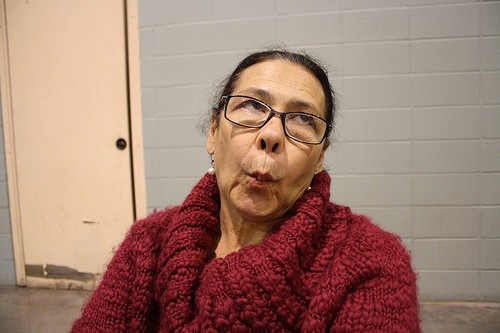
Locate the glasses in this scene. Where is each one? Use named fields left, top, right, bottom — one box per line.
left=221, top=94, right=333, bottom=146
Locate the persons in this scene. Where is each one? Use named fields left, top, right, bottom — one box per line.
left=69, top=48, right=422, bottom=333
